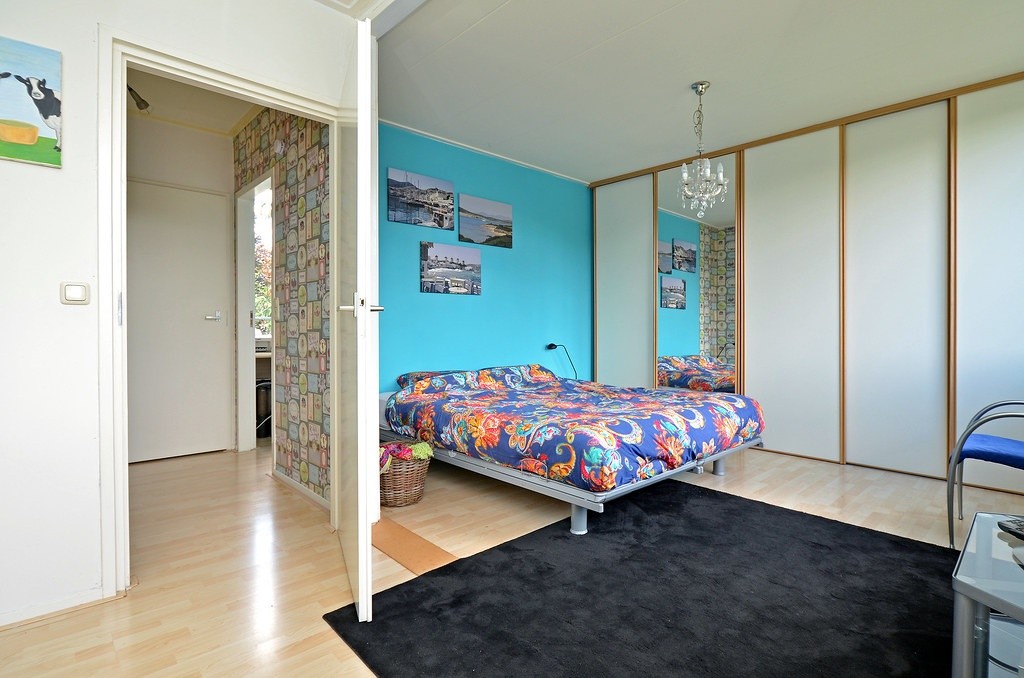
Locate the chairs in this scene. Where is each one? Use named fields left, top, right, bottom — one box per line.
left=946, top=400, right=1024, bottom=549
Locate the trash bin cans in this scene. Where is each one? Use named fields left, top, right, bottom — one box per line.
left=256, top=378, right=272, bottom=438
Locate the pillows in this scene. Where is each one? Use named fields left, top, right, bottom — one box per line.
left=397, top=370, right=465, bottom=390
left=380, top=366, right=766, bottom=535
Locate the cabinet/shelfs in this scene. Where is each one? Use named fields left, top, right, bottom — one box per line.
left=592, top=171, right=655, bottom=388
left=739, top=78, right=1024, bottom=494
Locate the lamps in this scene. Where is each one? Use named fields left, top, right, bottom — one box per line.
left=717, top=343, right=735, bottom=358
left=127, top=82, right=153, bottom=116
left=548, top=343, right=578, bottom=380
left=675, top=80, right=730, bottom=219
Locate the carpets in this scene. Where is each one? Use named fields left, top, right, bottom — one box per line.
left=323, top=476, right=1024, bottom=678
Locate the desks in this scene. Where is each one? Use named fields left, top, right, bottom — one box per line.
left=952, top=510, right=1024, bottom=678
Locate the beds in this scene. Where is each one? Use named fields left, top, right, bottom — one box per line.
left=658, top=355, right=735, bottom=392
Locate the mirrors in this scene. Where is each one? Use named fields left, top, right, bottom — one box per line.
left=654, top=152, right=735, bottom=395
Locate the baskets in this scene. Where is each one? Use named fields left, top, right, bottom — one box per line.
left=379, top=427, right=434, bottom=507
